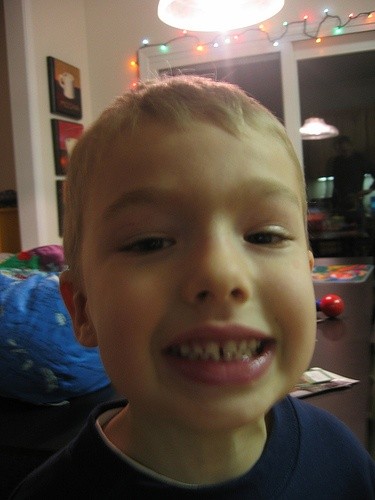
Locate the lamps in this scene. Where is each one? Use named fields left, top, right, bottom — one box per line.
left=300, top=117, right=340, bottom=141
left=157, top=0, right=284, bottom=31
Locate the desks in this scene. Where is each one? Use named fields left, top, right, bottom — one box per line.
left=289, top=256, right=375, bottom=462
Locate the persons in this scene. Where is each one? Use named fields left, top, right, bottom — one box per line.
left=9, top=71, right=375, bottom=499
left=329, top=135, right=375, bottom=257
